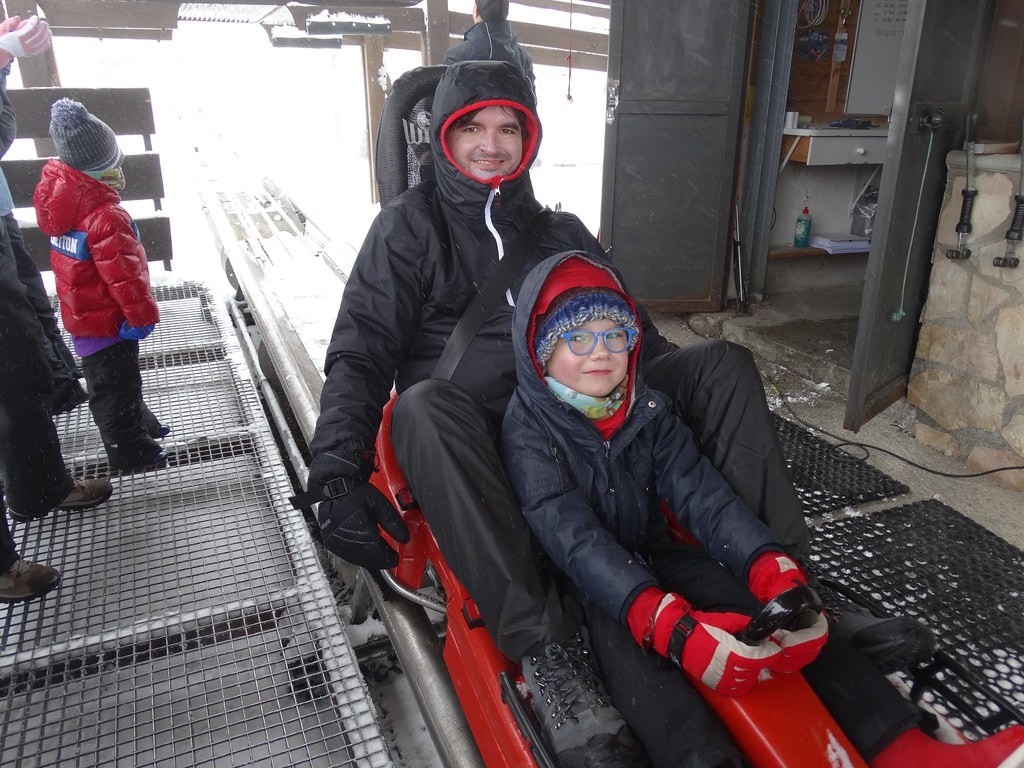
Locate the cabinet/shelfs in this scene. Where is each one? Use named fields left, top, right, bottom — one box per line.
left=767, top=123, right=889, bottom=259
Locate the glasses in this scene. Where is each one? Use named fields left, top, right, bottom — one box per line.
left=557, top=326, right=636, bottom=356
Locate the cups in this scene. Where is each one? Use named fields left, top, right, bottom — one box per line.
left=785, top=111, right=799, bottom=128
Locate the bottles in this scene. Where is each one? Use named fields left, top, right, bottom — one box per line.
left=794, top=206, right=812, bottom=248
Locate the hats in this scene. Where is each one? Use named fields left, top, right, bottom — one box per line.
left=533, top=287, right=640, bottom=375
left=49, top=99, right=125, bottom=175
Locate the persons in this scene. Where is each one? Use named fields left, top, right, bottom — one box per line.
left=444, top=0, right=537, bottom=86
left=308, top=63, right=937, bottom=768
left=0, top=15, right=113, bottom=604
left=499, top=251, right=830, bottom=768
left=34, top=98, right=171, bottom=464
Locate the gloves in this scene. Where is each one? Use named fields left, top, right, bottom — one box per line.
left=119, top=317, right=155, bottom=341
left=748, top=549, right=830, bottom=675
left=650, top=592, right=786, bottom=696
left=288, top=452, right=412, bottom=568
left=0, top=14, right=53, bottom=71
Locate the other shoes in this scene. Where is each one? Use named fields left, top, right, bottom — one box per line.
left=868, top=725, right=1024, bottom=768
left=108, top=447, right=169, bottom=472
left=153, top=424, right=170, bottom=439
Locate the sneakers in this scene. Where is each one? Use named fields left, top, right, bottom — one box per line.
left=515, top=627, right=646, bottom=768
left=804, top=572, right=936, bottom=675
left=0, top=556, right=62, bottom=604
left=9, top=478, right=114, bottom=522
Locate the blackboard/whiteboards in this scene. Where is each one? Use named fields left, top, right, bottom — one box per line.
left=844, top=0, right=908, bottom=117
